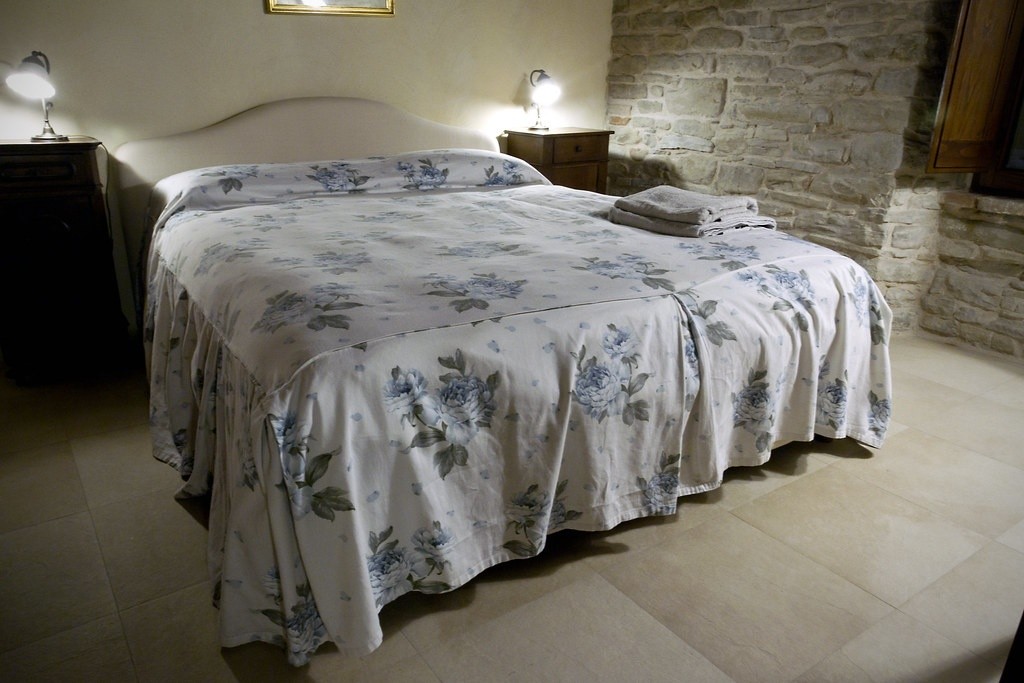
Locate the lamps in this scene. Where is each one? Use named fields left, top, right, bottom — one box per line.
left=528, top=69, right=558, bottom=130
left=5, top=50, right=69, bottom=142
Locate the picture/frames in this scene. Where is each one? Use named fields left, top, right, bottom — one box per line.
left=266, top=0, right=397, bottom=17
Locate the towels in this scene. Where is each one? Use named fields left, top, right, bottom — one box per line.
left=608, top=184, right=778, bottom=239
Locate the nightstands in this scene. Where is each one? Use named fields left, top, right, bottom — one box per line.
left=508, top=130, right=611, bottom=191
left=0, top=136, right=136, bottom=360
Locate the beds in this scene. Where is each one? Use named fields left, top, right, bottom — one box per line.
left=108, top=96, right=895, bottom=664
